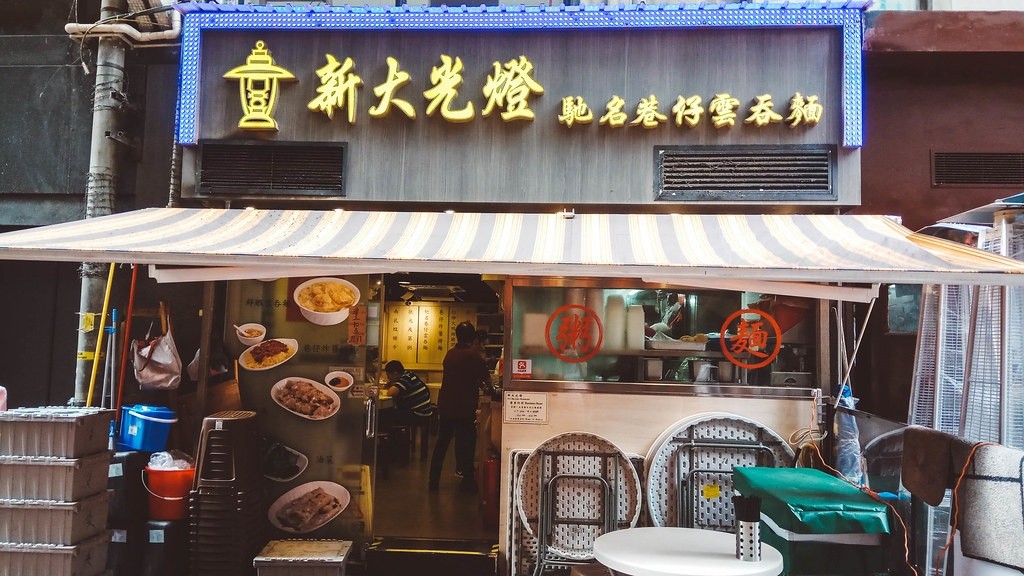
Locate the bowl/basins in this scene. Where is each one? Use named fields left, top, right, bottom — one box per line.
left=325, top=371, right=354, bottom=391
left=236, top=323, right=266, bottom=346
left=293, top=277, right=361, bottom=326
left=563, top=288, right=645, bottom=351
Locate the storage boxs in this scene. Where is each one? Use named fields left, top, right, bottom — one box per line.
left=0, top=488, right=116, bottom=546
left=0, top=529, right=114, bottom=576
left=119, top=405, right=174, bottom=453
left=0, top=405, right=117, bottom=459
left=0, top=456, right=117, bottom=502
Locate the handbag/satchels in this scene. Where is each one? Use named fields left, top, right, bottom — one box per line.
left=130, top=301, right=182, bottom=390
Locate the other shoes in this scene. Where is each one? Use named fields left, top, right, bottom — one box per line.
left=461, top=478, right=474, bottom=487
left=455, top=468, right=465, bottom=478
left=429, top=478, right=439, bottom=494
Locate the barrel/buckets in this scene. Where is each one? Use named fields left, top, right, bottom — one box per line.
left=142, top=466, right=196, bottom=521
left=117, top=403, right=179, bottom=453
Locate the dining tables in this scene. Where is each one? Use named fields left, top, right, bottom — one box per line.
left=366, top=395, right=392, bottom=409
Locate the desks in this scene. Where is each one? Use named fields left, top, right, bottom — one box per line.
left=505, top=410, right=889, bottom=576
left=519, top=346, right=753, bottom=385
left=480, top=401, right=502, bottom=460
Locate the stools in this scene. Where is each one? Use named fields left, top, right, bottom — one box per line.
left=476, top=457, right=500, bottom=524
left=188, top=411, right=260, bottom=576
left=408, top=423, right=428, bottom=462
left=388, top=428, right=410, bottom=470
left=370, top=433, right=390, bottom=481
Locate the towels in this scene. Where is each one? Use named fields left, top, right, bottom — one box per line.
left=900, top=428, right=981, bottom=508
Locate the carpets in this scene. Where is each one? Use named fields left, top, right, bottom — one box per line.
left=949, top=435, right=1024, bottom=574
left=900, top=426, right=957, bottom=507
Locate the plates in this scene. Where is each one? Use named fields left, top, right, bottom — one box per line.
left=263, top=445, right=307, bottom=483
left=268, top=480, right=350, bottom=534
left=238, top=338, right=298, bottom=371
left=271, top=377, right=341, bottom=420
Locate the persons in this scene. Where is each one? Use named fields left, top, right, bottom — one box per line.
left=428, top=321, right=494, bottom=496
left=382, top=360, right=433, bottom=426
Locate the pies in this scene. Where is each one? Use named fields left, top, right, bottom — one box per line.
left=276, top=487, right=335, bottom=527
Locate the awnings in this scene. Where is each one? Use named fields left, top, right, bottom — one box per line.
left=0, top=205, right=1024, bottom=297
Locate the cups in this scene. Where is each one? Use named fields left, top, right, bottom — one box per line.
left=693, top=362, right=711, bottom=382
left=718, top=362, right=732, bottom=382
left=647, top=360, right=662, bottom=380
left=688, top=360, right=702, bottom=378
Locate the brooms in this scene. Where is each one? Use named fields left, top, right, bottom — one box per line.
left=108, top=307, right=117, bottom=450
